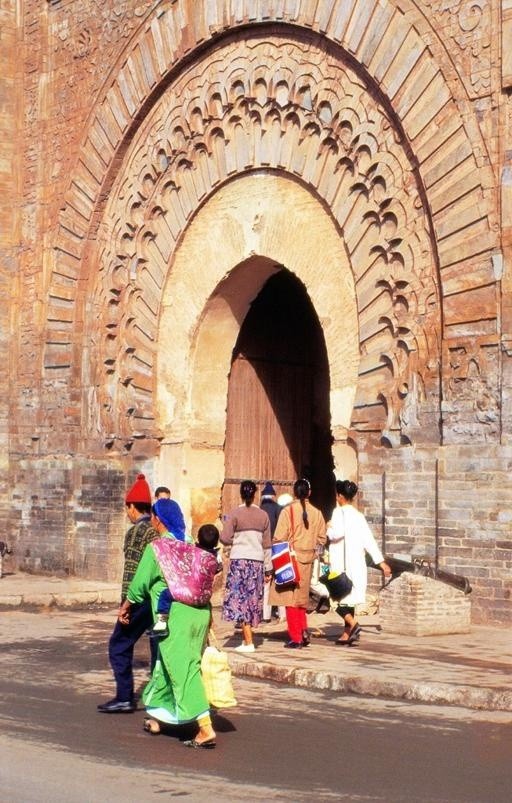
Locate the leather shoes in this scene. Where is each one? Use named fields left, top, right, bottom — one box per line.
left=96, top=697, right=137, bottom=712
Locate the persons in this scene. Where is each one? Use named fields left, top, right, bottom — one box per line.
left=219, top=481, right=274, bottom=653
left=258, top=478, right=391, bottom=649
left=118, top=499, right=217, bottom=749
left=98, top=474, right=161, bottom=713
left=155, top=487, right=171, bottom=502
left=145, top=524, right=221, bottom=638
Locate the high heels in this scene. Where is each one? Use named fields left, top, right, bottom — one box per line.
left=335, top=621, right=362, bottom=646
left=284, top=629, right=310, bottom=649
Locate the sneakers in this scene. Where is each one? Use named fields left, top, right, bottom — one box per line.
left=234, top=639, right=255, bottom=652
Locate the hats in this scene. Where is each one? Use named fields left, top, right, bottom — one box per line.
left=261, top=481, right=277, bottom=495
left=277, top=493, right=293, bottom=506
left=126, top=473, right=151, bottom=503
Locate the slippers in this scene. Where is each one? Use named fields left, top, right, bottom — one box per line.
left=143, top=717, right=162, bottom=733
left=183, top=738, right=217, bottom=748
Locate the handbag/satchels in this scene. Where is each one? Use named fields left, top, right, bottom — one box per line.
left=319, top=571, right=352, bottom=602
left=271, top=542, right=300, bottom=588
left=200, top=628, right=238, bottom=708
